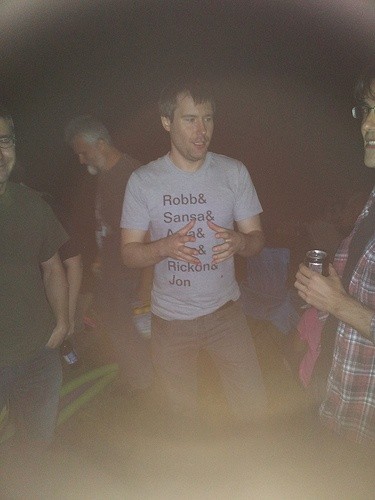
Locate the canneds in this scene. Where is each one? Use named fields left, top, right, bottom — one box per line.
left=303, top=249, right=330, bottom=276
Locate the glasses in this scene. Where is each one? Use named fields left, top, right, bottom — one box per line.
left=0, top=136, right=16, bottom=149
left=351, top=103, right=375, bottom=120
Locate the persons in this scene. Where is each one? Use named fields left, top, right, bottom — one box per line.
left=117, top=80, right=282, bottom=500
left=1, top=111, right=144, bottom=500
left=291, top=60, right=374, bottom=500
